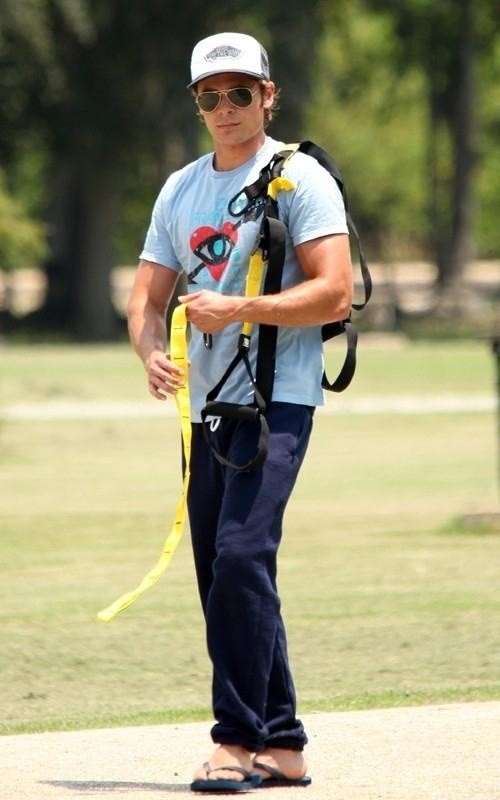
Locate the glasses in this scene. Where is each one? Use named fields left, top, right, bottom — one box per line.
left=199, top=85, right=253, bottom=113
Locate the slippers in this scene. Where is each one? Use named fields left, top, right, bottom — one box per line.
left=252, top=754, right=313, bottom=787
left=190, top=758, right=262, bottom=791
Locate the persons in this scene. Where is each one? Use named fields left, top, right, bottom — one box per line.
left=126, top=28, right=356, bottom=791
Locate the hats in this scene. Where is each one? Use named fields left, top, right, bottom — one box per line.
left=186, top=28, right=274, bottom=92
